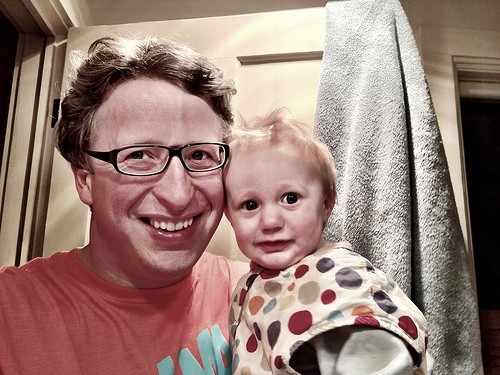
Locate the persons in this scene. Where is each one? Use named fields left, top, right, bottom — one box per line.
left=0, top=33, right=251, bottom=375
left=219, top=105, right=436, bottom=375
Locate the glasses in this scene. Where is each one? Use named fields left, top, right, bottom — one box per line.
left=82, top=142, right=229, bottom=177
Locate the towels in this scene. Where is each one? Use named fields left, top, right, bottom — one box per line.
left=311, top=0, right=486, bottom=375
